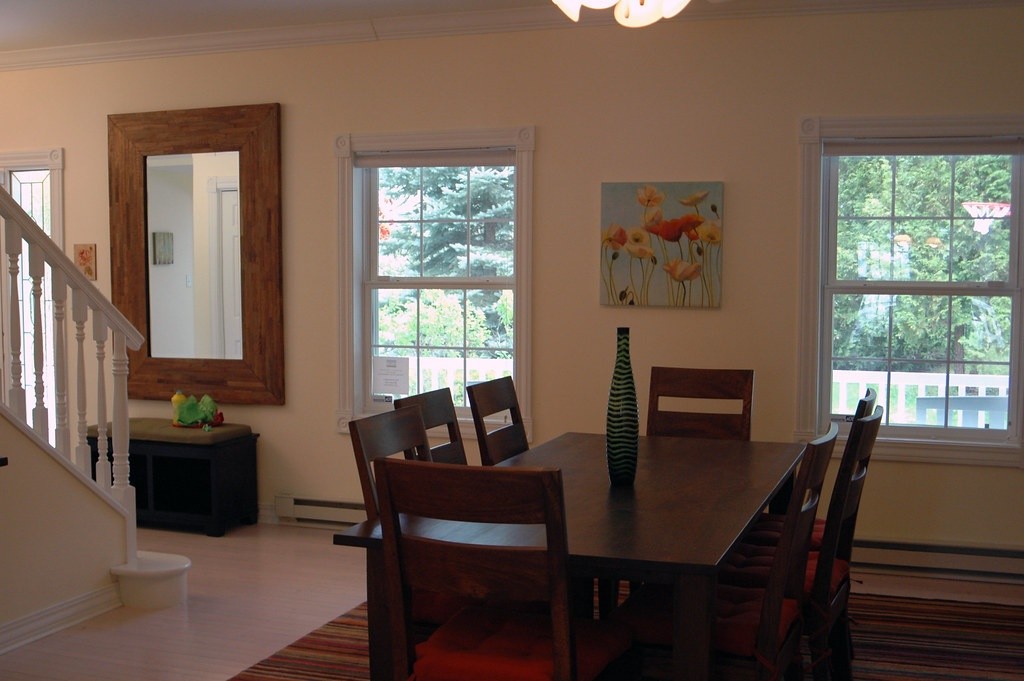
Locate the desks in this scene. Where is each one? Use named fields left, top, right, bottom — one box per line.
left=333, top=432, right=811, bottom=681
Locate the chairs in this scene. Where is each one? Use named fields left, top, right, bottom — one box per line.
left=610, top=366, right=883, bottom=681
left=372, top=455, right=638, bottom=681
left=348, top=404, right=453, bottom=627
left=468, top=376, right=529, bottom=466
left=394, top=387, right=468, bottom=465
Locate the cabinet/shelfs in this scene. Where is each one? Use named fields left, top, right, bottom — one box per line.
left=87, top=416, right=259, bottom=536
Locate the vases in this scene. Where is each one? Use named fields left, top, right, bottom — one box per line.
left=607, top=325, right=639, bottom=487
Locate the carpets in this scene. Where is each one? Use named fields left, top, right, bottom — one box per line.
left=224, top=593, right=1024, bottom=681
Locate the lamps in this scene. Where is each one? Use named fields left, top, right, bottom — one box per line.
left=549, top=0, right=691, bottom=27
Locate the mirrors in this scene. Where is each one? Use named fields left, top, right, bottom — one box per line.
left=106, top=102, right=286, bottom=406
